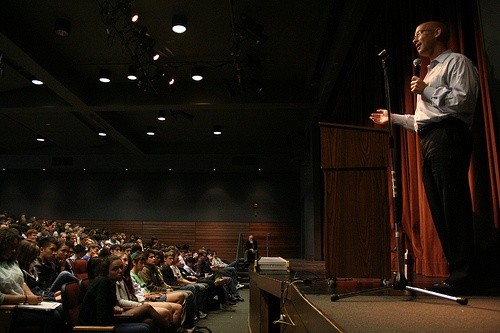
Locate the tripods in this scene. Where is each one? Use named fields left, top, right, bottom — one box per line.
left=330, top=49, right=467, bottom=305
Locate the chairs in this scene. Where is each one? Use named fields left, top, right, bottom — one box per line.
left=0, top=259, right=115, bottom=333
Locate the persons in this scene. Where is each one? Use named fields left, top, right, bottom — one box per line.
left=0, top=215, right=245, bottom=333
left=369, top=22, right=482, bottom=293
left=245, top=234, right=258, bottom=260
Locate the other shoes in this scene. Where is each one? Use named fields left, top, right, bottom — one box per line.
left=226, top=295, right=244, bottom=305
left=178, top=327, right=196, bottom=333
left=220, top=303, right=236, bottom=312
left=243, top=263, right=247, bottom=268
left=423, top=279, right=484, bottom=297
left=194, top=310, right=208, bottom=323
left=236, top=283, right=244, bottom=290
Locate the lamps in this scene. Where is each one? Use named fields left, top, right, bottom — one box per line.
left=54, top=0, right=205, bottom=86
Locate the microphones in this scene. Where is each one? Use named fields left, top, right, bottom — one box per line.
left=413, top=58, right=422, bottom=77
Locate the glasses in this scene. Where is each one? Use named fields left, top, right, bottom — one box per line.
left=414, top=29, right=433, bottom=37
left=149, top=256, right=156, bottom=258
